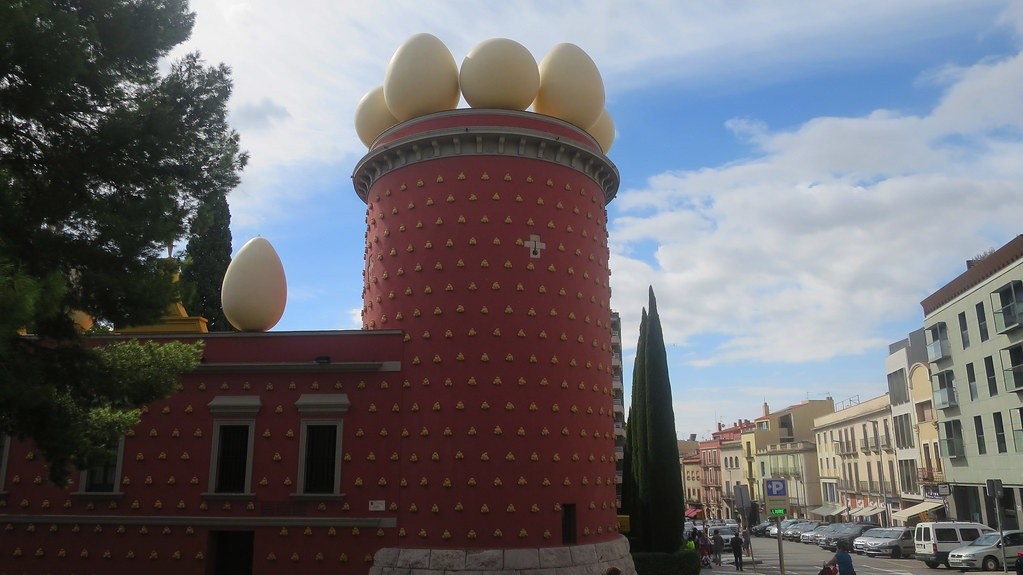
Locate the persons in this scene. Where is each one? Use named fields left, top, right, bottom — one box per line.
left=742, top=527, right=750, bottom=557
left=823, top=540, right=856, bottom=575
left=686, top=528, right=725, bottom=566
left=730, top=533, right=743, bottom=571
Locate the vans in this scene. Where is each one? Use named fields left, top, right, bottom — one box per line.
left=913, top=520, right=996, bottom=570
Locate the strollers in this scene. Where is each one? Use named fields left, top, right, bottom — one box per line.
left=816, top=560, right=839, bottom=575
left=700, top=542, right=713, bottom=569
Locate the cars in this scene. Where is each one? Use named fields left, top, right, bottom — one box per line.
left=750, top=517, right=881, bottom=553
left=853, top=526, right=889, bottom=556
left=683, top=517, right=740, bottom=554
left=945, top=530, right=1023, bottom=571
left=864, top=526, right=919, bottom=559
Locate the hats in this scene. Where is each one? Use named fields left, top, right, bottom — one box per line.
left=836, top=539, right=849, bottom=550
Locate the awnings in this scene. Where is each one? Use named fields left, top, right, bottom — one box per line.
left=685, top=509, right=702, bottom=518
left=810, top=505, right=847, bottom=517
left=841, top=506, right=886, bottom=517
left=891, top=502, right=945, bottom=522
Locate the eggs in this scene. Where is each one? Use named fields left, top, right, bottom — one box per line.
left=222, top=237, right=287, bottom=332
left=354, top=33, right=615, bottom=154
